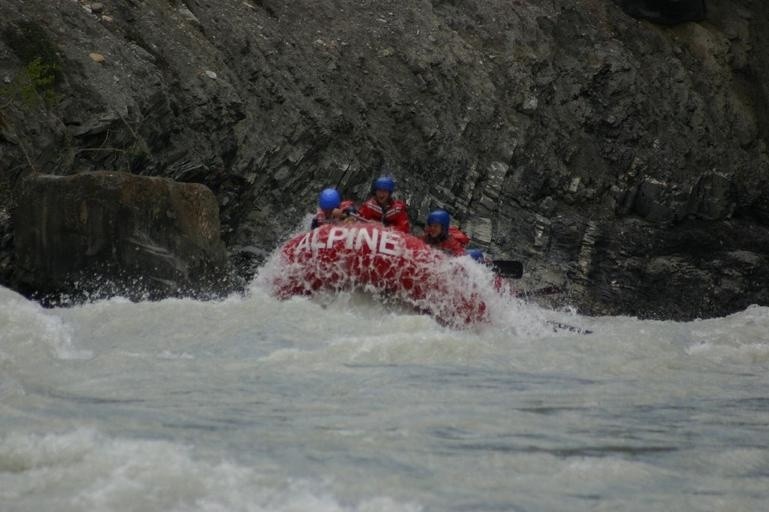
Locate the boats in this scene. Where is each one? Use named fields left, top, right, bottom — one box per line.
left=269, top=223, right=516, bottom=335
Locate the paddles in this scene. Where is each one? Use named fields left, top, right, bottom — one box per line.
left=514, top=285, right=561, bottom=298
left=491, top=260, right=523, bottom=279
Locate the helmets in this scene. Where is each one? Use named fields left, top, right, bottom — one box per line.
left=464, top=249, right=484, bottom=263
left=374, top=176, right=395, bottom=192
left=319, top=188, right=340, bottom=209
left=427, top=210, right=450, bottom=225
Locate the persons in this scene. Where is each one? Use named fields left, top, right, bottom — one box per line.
left=301, top=175, right=492, bottom=269
